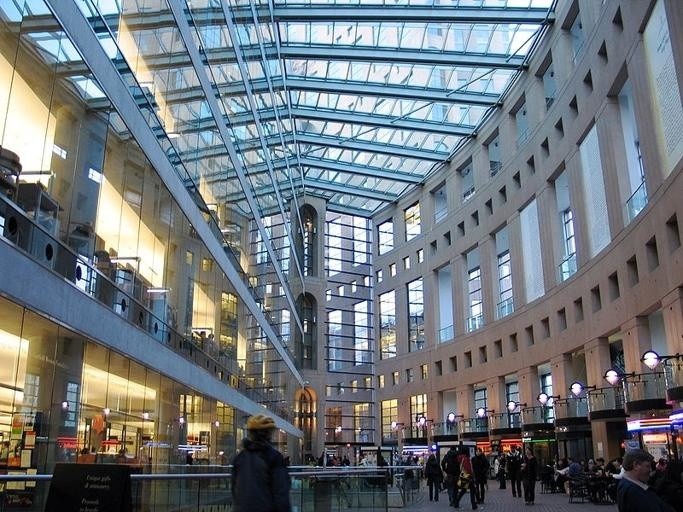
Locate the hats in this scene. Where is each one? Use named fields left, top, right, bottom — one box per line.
left=596, top=457, right=605, bottom=462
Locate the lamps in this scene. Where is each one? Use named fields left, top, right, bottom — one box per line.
left=505, top=400, right=527, bottom=412
left=571, top=376, right=596, bottom=396
left=419, top=416, right=434, bottom=425
left=537, top=391, right=560, bottom=406
left=604, top=366, right=636, bottom=386
left=641, top=350, right=683, bottom=372
left=477, top=407, right=494, bottom=419
left=391, top=421, right=404, bottom=428
left=448, top=412, right=464, bottom=422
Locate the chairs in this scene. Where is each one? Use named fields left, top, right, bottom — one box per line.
left=541, top=469, right=620, bottom=505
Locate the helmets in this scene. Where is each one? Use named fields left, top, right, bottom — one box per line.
left=246, top=413, right=277, bottom=430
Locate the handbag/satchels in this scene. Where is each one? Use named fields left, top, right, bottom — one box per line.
left=520, top=455, right=527, bottom=474
left=456, top=457, right=475, bottom=489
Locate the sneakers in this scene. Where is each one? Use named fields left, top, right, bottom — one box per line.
left=472, top=498, right=484, bottom=511
left=449, top=499, right=459, bottom=508
left=524, top=501, right=536, bottom=506
left=429, top=497, right=439, bottom=502
left=498, top=486, right=507, bottom=490
left=513, top=493, right=522, bottom=498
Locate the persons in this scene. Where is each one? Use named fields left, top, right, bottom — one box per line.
left=76, top=446, right=209, bottom=464
left=494, top=448, right=537, bottom=506
left=617, top=449, right=683, bottom=512
left=358, top=455, right=371, bottom=489
left=393, top=451, right=425, bottom=473
left=0, top=437, right=11, bottom=461
left=230, top=413, right=292, bottom=511
left=377, top=455, right=389, bottom=491
left=539, top=457, right=625, bottom=504
left=309, top=454, right=351, bottom=490
left=175, top=451, right=229, bottom=490
left=425, top=446, right=490, bottom=511
